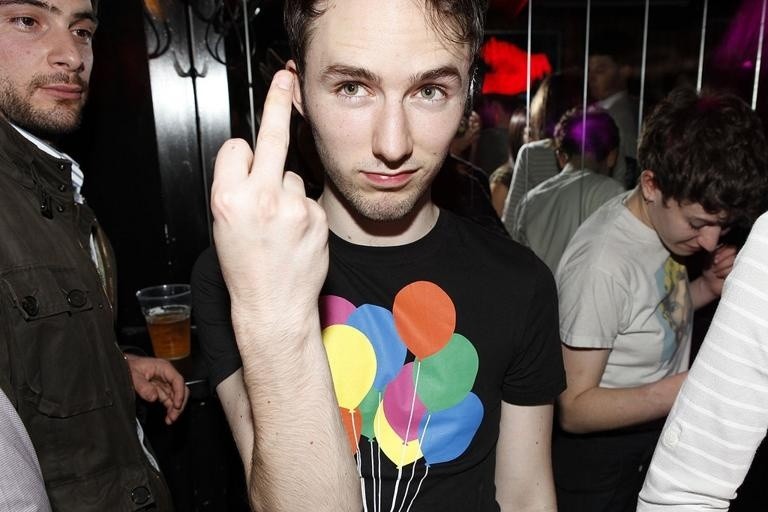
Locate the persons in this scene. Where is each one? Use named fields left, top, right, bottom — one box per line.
left=2, top=1, right=190, bottom=511
left=636, top=210, right=768, bottom=512
left=193, top=1, right=567, bottom=512
left=550, top=84, right=768, bottom=512
left=444, top=47, right=639, bottom=280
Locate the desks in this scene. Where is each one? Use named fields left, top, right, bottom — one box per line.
left=111, top=322, right=223, bottom=413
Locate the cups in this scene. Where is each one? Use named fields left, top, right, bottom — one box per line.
left=137, top=282, right=194, bottom=361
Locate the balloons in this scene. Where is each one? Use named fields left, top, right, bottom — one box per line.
left=313, top=279, right=485, bottom=470
